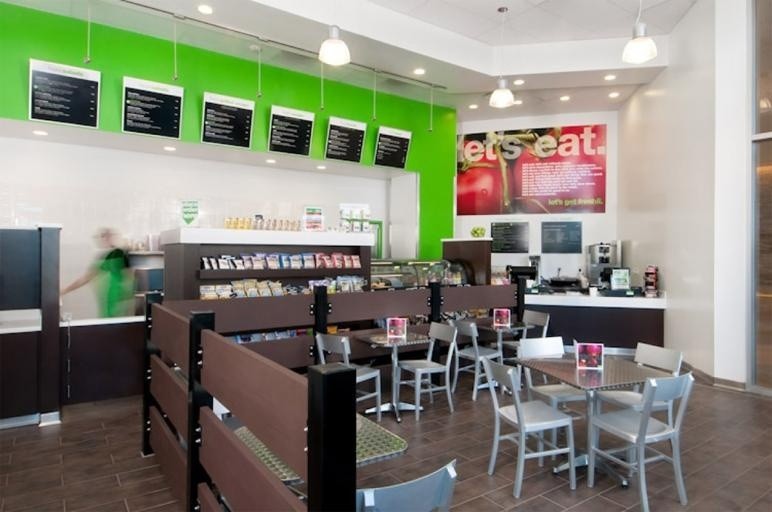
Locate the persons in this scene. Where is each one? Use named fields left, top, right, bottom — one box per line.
left=60, top=226, right=136, bottom=318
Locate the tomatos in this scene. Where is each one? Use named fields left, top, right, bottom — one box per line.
left=457, top=140, right=513, bottom=214
left=514, top=125, right=606, bottom=213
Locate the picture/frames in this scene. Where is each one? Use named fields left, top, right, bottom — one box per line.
left=493, top=309, right=511, bottom=326
left=387, top=318, right=408, bottom=338
left=573, top=343, right=604, bottom=372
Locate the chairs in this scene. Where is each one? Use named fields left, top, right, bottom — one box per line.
left=479, top=358, right=577, bottom=499
left=392, top=322, right=459, bottom=420
left=314, top=334, right=383, bottom=428
left=501, top=309, right=550, bottom=389
left=357, top=460, right=461, bottom=512
left=451, top=320, right=503, bottom=401
left=589, top=342, right=685, bottom=462
left=587, top=372, right=693, bottom=512
left=518, top=336, right=591, bottom=461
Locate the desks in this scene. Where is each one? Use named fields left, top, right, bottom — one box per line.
left=351, top=325, right=433, bottom=412
left=466, top=315, right=535, bottom=396
left=509, top=355, right=677, bottom=490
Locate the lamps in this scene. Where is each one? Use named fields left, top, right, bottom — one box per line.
left=489, top=78, right=515, bottom=109
left=318, top=25, right=350, bottom=66
left=623, top=23, right=657, bottom=64
